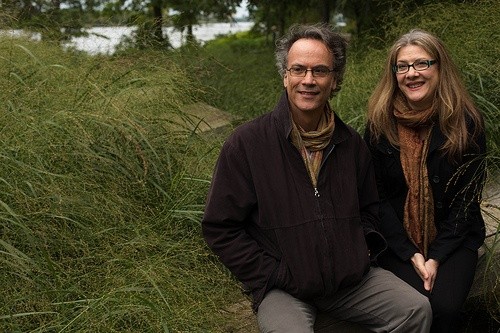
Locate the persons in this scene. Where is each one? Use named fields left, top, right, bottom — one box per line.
left=363, top=31, right=487, bottom=333
left=201, top=25, right=433, bottom=333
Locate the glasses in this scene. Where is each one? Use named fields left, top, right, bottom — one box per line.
left=286, top=66, right=335, bottom=78
left=393, top=60, right=437, bottom=74
